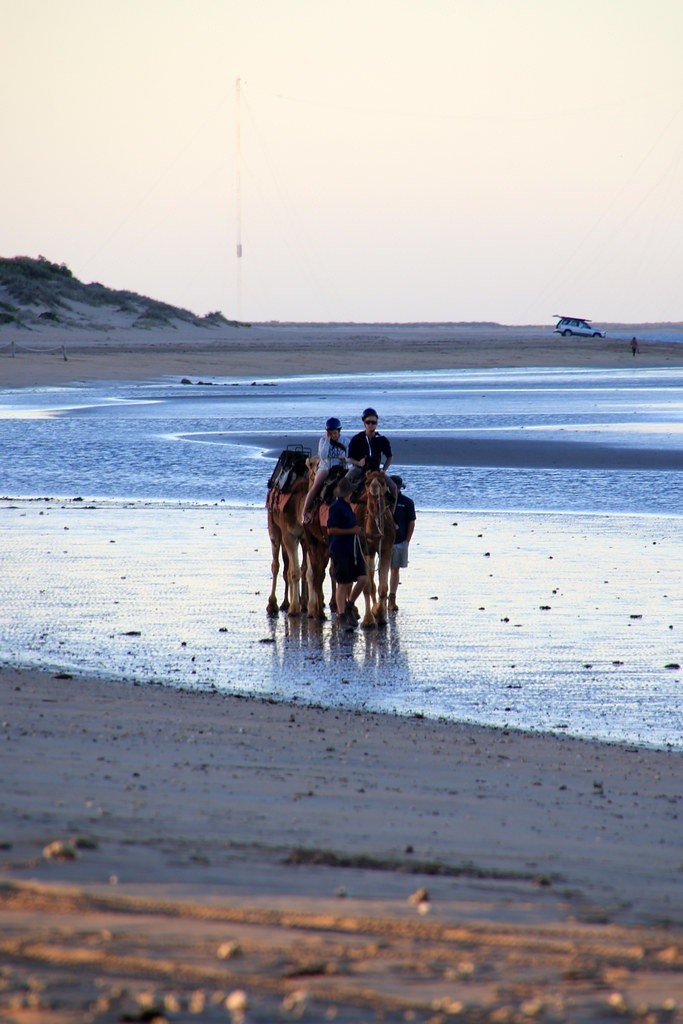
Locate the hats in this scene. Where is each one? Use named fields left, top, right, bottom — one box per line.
left=333, top=476, right=358, bottom=498
left=362, top=408, right=378, bottom=422
left=389, top=475, right=406, bottom=489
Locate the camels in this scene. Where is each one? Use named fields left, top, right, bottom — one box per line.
left=265, top=455, right=397, bottom=630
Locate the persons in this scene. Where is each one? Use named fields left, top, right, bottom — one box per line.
left=345, top=406, right=400, bottom=531
left=301, top=417, right=351, bottom=524
left=324, top=475, right=368, bottom=634
left=630, top=336, right=639, bottom=356
left=374, top=475, right=416, bottom=614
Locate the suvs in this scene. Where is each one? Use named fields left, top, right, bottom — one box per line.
left=552, top=314, right=606, bottom=338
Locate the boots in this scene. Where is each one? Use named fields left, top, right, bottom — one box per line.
left=387, top=594, right=398, bottom=611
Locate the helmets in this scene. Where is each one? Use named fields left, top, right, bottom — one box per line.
left=326, top=417, right=342, bottom=431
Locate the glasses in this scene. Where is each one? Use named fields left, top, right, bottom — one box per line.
left=365, top=420, right=377, bottom=425
